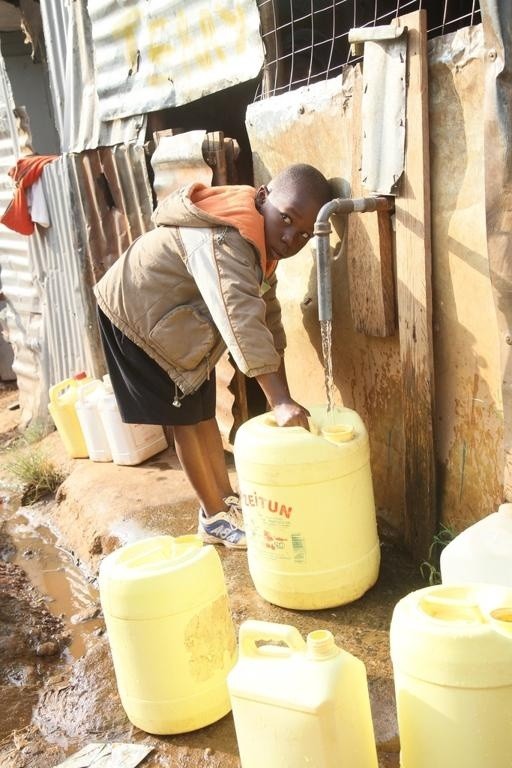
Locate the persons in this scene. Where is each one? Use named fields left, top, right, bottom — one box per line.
left=92, top=165, right=334, bottom=551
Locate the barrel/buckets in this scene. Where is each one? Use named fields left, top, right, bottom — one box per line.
left=440, top=503, right=512, bottom=586
left=97, top=390, right=169, bottom=466
left=47, top=372, right=91, bottom=458
left=97, top=533, right=238, bottom=732
left=233, top=405, right=380, bottom=609
left=75, top=373, right=112, bottom=462
left=226, top=621, right=378, bottom=767
left=388, top=585, right=512, bottom=767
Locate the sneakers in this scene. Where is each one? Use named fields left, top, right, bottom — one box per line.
left=197, top=495, right=254, bottom=548
